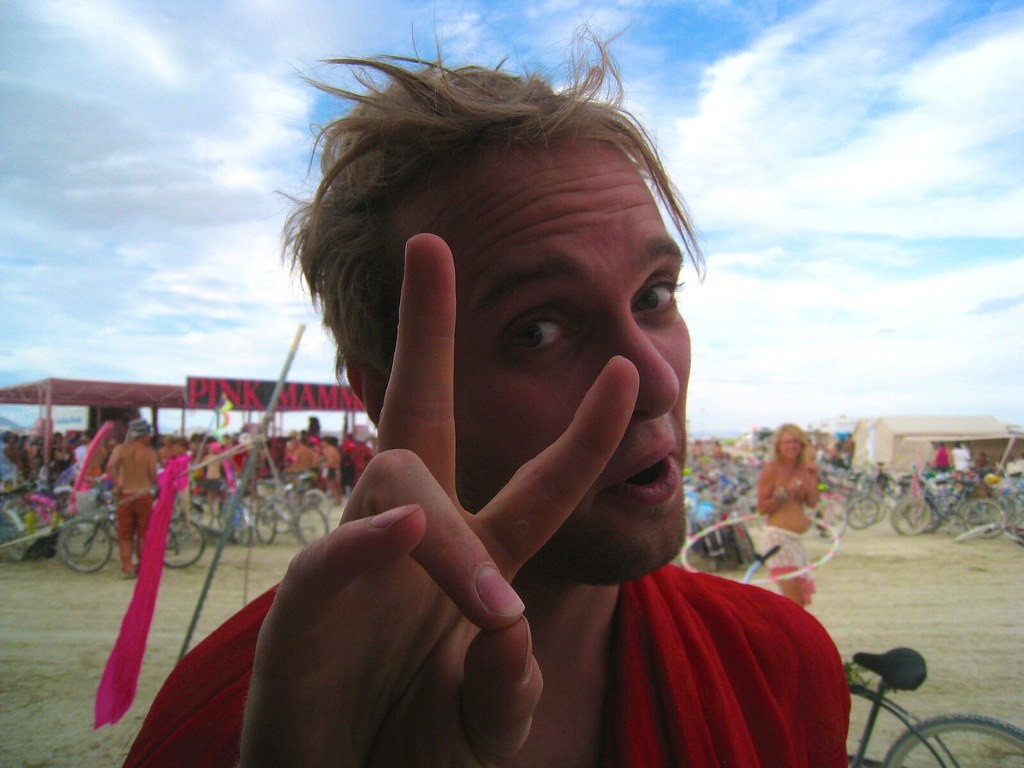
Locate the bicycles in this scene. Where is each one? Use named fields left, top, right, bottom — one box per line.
left=680, top=476, right=757, bottom=572
left=0, top=474, right=331, bottom=574
left=818, top=461, right=1024, bottom=544
left=743, top=545, right=1024, bottom=768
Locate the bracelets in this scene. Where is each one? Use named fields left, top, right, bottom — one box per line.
left=774, top=486, right=788, bottom=499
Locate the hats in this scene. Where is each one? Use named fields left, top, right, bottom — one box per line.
left=128, top=417, right=150, bottom=439
left=206, top=442, right=222, bottom=454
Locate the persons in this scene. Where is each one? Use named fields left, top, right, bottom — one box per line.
left=148, top=423, right=377, bottom=506
left=692, top=440, right=703, bottom=458
left=106, top=418, right=158, bottom=579
left=0, top=427, right=118, bottom=496
left=121, top=49, right=851, bottom=768
left=828, top=434, right=855, bottom=467
left=756, top=422, right=819, bottom=608
left=712, top=441, right=722, bottom=457
left=934, top=441, right=989, bottom=476
